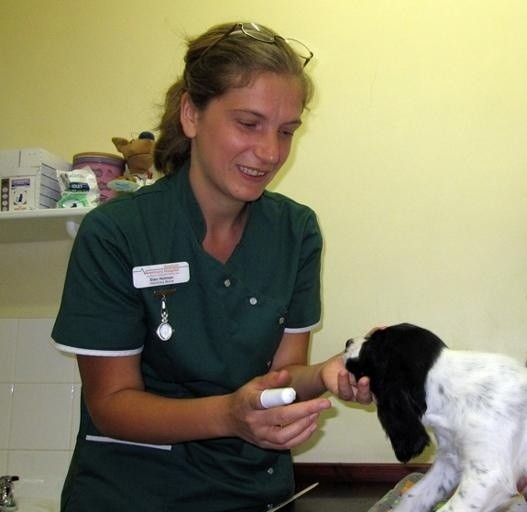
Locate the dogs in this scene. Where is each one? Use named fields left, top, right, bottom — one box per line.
left=342, top=321, right=527, bottom=512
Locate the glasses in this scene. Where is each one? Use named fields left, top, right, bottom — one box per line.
left=191, top=21, right=314, bottom=70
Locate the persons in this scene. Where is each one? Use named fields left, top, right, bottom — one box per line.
left=52, top=19, right=376, bottom=510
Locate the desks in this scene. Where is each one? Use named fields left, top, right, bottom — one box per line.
left=361, top=473, right=527, bottom=512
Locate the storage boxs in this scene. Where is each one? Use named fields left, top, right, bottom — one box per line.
left=0, top=148, right=72, bottom=212
